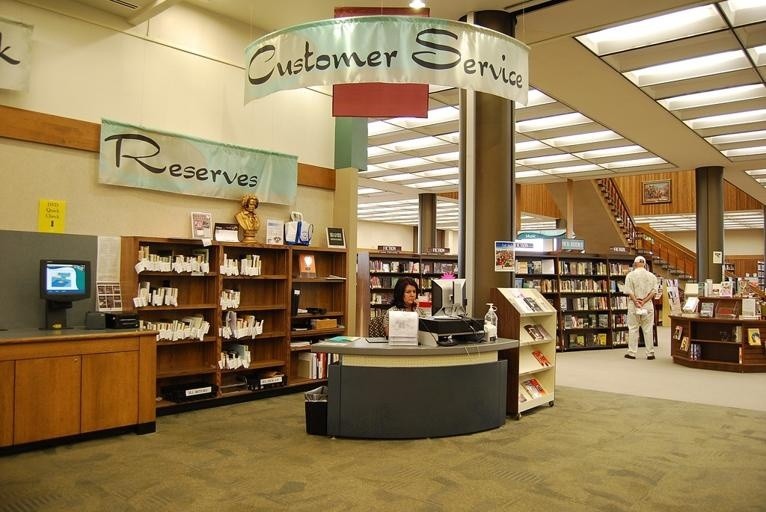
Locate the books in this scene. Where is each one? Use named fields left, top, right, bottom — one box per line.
left=311, top=319, right=337, bottom=330
left=532, top=351, right=553, bottom=368
left=742, top=299, right=755, bottom=316
left=609, top=278, right=625, bottom=292
left=721, top=325, right=742, bottom=344
left=222, top=312, right=263, bottom=337
left=609, top=262, right=632, bottom=275
left=221, top=288, right=241, bottom=309
left=174, top=249, right=209, bottom=273
left=699, top=278, right=713, bottom=297
left=535, top=325, right=552, bottom=340
left=224, top=252, right=262, bottom=276
left=683, top=296, right=700, bottom=313
left=139, top=312, right=210, bottom=341
left=558, top=261, right=607, bottom=276
left=655, top=278, right=664, bottom=299
left=299, top=253, right=316, bottom=280
left=422, top=277, right=439, bottom=288
left=516, top=257, right=555, bottom=274
left=139, top=280, right=178, bottom=306
left=610, top=296, right=629, bottom=309
left=290, top=341, right=310, bottom=348
left=523, top=325, right=543, bottom=340
left=424, top=259, right=458, bottom=273
left=524, top=297, right=543, bottom=312
left=325, top=335, right=363, bottom=343
left=370, top=276, right=420, bottom=288
left=672, top=326, right=682, bottom=340
left=546, top=295, right=557, bottom=307
left=214, top=223, right=240, bottom=241
left=220, top=344, right=251, bottom=369
left=564, top=332, right=607, bottom=347
left=613, top=331, right=629, bottom=344
left=369, top=259, right=420, bottom=274
left=559, top=278, right=608, bottom=293
left=739, top=345, right=742, bottom=364
left=366, top=337, right=388, bottom=342
left=748, top=328, right=761, bottom=346
left=611, top=313, right=627, bottom=327
left=138, top=246, right=171, bottom=271
left=757, top=261, right=766, bottom=292
left=719, top=281, right=733, bottom=296
left=370, top=308, right=386, bottom=317
left=560, top=297, right=607, bottom=311
left=371, top=290, right=395, bottom=304
left=292, top=283, right=299, bottom=317
left=680, top=336, right=690, bottom=351
left=515, top=277, right=557, bottom=291
left=723, top=263, right=735, bottom=281
left=700, top=303, right=713, bottom=317
left=561, top=313, right=608, bottom=328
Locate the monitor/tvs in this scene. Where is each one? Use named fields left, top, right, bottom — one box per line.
left=431, top=279, right=467, bottom=318
left=39, top=259, right=91, bottom=330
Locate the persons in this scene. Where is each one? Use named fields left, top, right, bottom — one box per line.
left=383, top=277, right=427, bottom=341
left=623, top=256, right=658, bottom=360
left=640, top=326, right=641, bottom=326
left=235, top=194, right=261, bottom=244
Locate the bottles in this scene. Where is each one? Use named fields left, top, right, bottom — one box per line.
left=485, top=304, right=498, bottom=343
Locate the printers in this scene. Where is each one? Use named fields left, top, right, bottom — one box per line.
left=84, top=310, right=140, bottom=330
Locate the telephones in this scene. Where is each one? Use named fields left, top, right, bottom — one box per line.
left=411, top=301, right=417, bottom=312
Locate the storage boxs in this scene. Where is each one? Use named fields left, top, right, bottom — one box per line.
left=121, top=237, right=221, bottom=405
left=419, top=253, right=458, bottom=314
left=492, top=287, right=555, bottom=419
left=669, top=293, right=765, bottom=373
left=511, top=256, right=561, bottom=350
left=291, top=245, right=348, bottom=389
left=221, top=242, right=289, bottom=395
left=14, top=350, right=140, bottom=452
left=609, top=252, right=658, bottom=347
left=360, top=251, right=420, bottom=335
left=557, top=254, right=612, bottom=349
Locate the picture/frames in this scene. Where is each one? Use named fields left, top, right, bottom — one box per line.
left=494, top=240, right=515, bottom=272
left=326, top=228, right=346, bottom=249
left=95, top=281, right=122, bottom=312
left=640, top=179, right=672, bottom=204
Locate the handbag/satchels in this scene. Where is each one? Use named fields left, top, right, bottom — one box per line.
left=284, top=211, right=314, bottom=246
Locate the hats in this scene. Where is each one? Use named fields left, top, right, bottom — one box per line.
left=634, top=255, right=646, bottom=264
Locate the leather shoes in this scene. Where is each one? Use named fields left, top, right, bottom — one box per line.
left=624, top=354, right=636, bottom=359
left=646, top=355, right=656, bottom=360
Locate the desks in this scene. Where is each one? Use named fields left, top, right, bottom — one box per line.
left=311, top=334, right=518, bottom=439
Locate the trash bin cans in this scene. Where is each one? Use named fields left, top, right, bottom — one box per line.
left=304, top=385, right=329, bottom=437
